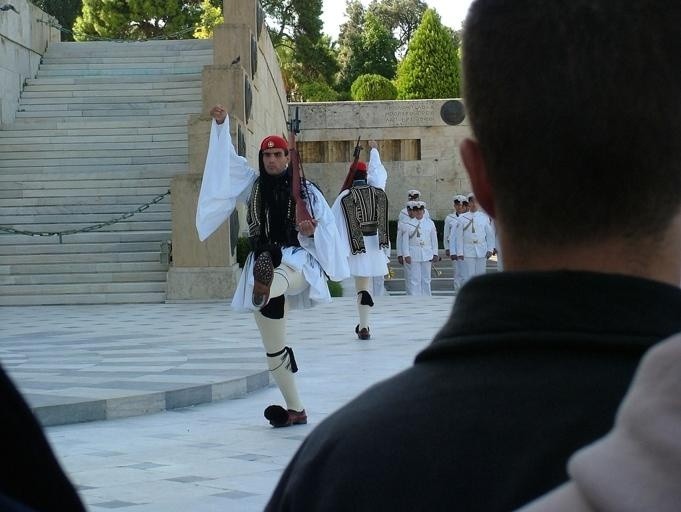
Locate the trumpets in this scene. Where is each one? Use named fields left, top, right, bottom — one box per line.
left=430, top=261, right=443, bottom=276
left=387, top=262, right=395, bottom=279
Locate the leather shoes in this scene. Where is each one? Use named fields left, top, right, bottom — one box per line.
left=252, top=243, right=282, bottom=307
left=356, top=324, right=370, bottom=339
left=265, top=406, right=308, bottom=427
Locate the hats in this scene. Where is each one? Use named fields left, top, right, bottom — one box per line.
left=405, top=190, right=426, bottom=209
left=261, top=137, right=287, bottom=151
left=351, top=161, right=367, bottom=171
left=454, top=192, right=475, bottom=204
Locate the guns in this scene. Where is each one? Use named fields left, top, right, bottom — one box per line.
left=339, top=137, right=364, bottom=193
left=291, top=108, right=311, bottom=226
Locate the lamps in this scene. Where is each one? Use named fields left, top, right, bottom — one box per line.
left=286, top=119, right=301, bottom=133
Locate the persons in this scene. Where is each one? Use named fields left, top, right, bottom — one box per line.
left=1, top=358, right=88, bottom=511
left=194, top=103, right=344, bottom=427
left=515, top=327, right=680, bottom=511
left=263, top=2, right=681, bottom=511
left=327, top=140, right=393, bottom=341
left=394, top=183, right=504, bottom=296
